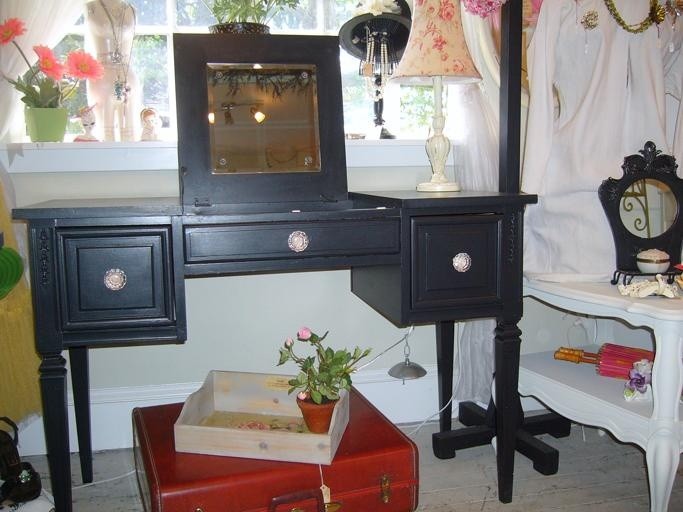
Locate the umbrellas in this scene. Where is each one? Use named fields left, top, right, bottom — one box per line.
left=552, top=348, right=649, bottom=380
left=557, top=341, right=657, bottom=365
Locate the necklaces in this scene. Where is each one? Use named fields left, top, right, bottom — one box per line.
left=359, top=21, right=392, bottom=102
left=97, top=1, right=138, bottom=108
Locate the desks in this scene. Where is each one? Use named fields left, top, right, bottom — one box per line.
left=7, top=34, right=538, bottom=511
left=490, top=275, right=682, bottom=512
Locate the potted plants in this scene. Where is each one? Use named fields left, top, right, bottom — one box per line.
left=298, top=394, right=340, bottom=433
left=198, top=1, right=299, bottom=35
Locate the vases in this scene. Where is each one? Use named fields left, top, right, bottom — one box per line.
left=21, top=105, right=70, bottom=143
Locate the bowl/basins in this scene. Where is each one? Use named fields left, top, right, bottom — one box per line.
left=636, top=249, right=670, bottom=273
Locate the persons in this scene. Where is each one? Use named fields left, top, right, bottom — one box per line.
left=71, top=109, right=101, bottom=143
left=136, top=107, right=161, bottom=143
left=84, top=1, right=139, bottom=145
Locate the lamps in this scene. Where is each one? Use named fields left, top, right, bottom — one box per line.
left=390, top=1, right=484, bottom=191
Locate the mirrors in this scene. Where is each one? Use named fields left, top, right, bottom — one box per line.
left=172, top=31, right=349, bottom=201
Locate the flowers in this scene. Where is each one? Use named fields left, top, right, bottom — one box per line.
left=0, top=18, right=101, bottom=107
left=280, top=323, right=370, bottom=402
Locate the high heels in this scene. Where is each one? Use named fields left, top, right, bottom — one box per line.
left=0, top=414, right=41, bottom=502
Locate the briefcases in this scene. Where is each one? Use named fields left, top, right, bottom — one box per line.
left=132, top=385, right=419, bottom=512
